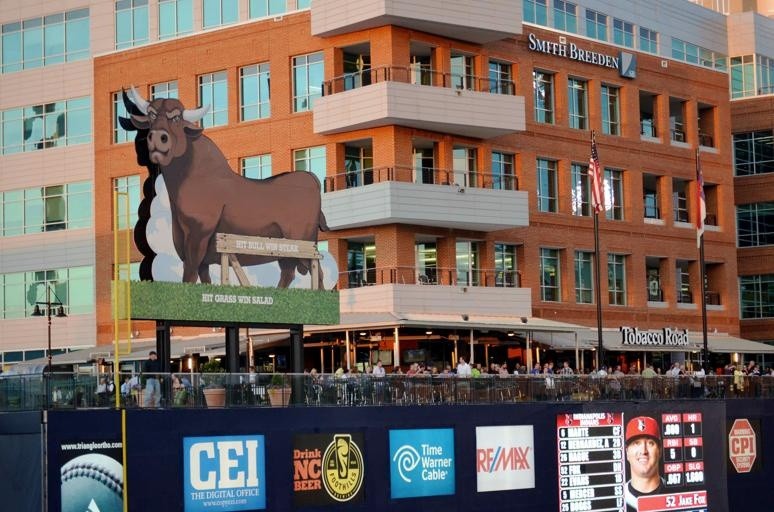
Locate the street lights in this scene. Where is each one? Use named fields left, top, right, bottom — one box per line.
left=31, top=287, right=67, bottom=370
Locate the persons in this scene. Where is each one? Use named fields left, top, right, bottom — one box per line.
left=304, top=355, right=527, bottom=406
left=249, top=367, right=259, bottom=387
left=532, top=360, right=705, bottom=401
left=52, top=351, right=181, bottom=409
left=625, top=417, right=665, bottom=511
left=706, top=360, right=773, bottom=399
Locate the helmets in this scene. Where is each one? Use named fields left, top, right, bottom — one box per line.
left=625, top=416, right=662, bottom=442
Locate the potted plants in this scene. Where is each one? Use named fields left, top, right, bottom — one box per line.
left=267, top=371, right=293, bottom=407
left=198, top=357, right=227, bottom=409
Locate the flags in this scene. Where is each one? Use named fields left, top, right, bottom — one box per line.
left=695, top=149, right=708, bottom=250
left=586, top=128, right=604, bottom=216
